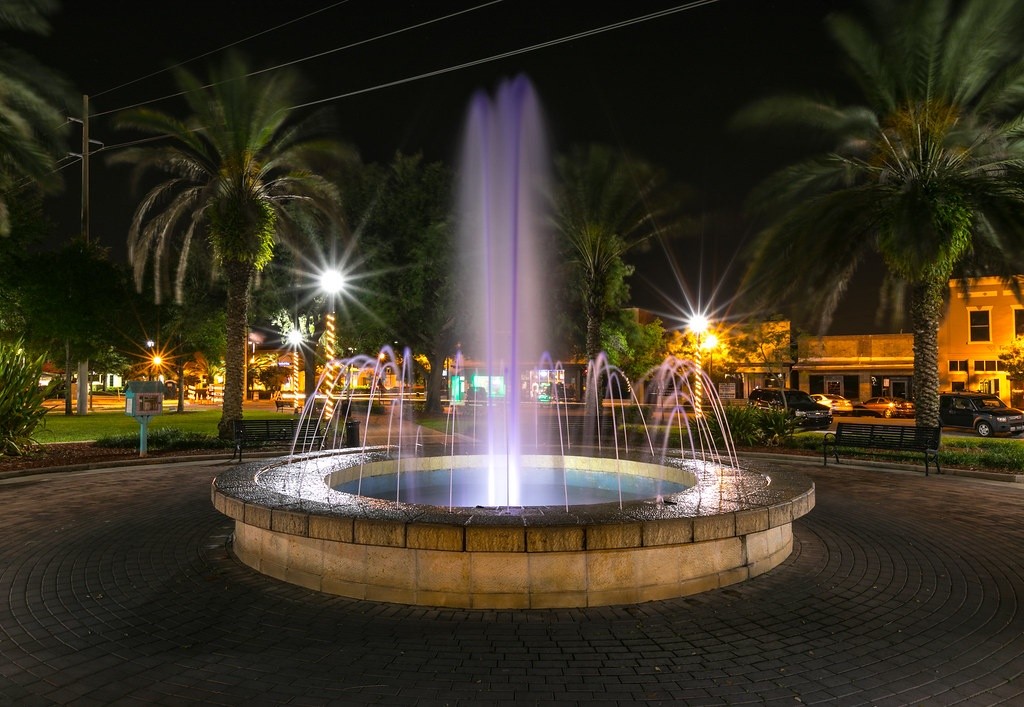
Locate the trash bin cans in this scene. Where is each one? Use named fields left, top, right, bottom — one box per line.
left=346, top=421, right=361, bottom=447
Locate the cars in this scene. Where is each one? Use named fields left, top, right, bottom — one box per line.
left=809, top=393, right=853, bottom=416
left=853, top=396, right=916, bottom=419
left=463, top=386, right=488, bottom=407
left=747, top=387, right=833, bottom=430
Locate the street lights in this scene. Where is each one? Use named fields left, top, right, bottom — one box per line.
left=318, top=267, right=344, bottom=419
left=287, top=327, right=302, bottom=410
left=686, top=314, right=710, bottom=418
left=145, top=339, right=156, bottom=381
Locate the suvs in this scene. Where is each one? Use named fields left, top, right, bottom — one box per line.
left=938, top=387, right=1024, bottom=436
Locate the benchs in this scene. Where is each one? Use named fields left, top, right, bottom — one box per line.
left=536, top=416, right=617, bottom=446
left=822, top=421, right=943, bottom=477
left=228, top=418, right=328, bottom=464
left=275, top=400, right=301, bottom=413
